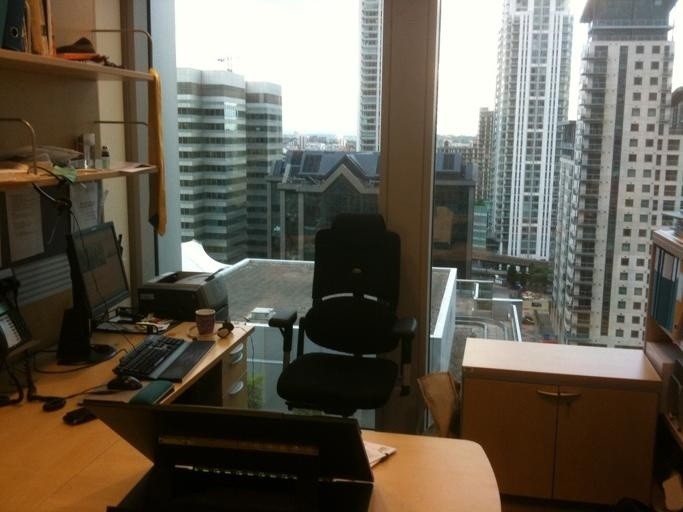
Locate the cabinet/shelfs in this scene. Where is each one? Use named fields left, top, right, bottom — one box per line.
left=453, top=330, right=663, bottom=512
left=0, top=47, right=163, bottom=192
left=643, top=225, right=683, bottom=512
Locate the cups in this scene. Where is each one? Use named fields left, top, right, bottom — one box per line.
left=195, top=309, right=214, bottom=333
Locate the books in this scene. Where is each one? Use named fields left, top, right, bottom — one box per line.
left=0, top=0, right=55, bottom=60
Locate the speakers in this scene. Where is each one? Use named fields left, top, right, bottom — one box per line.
left=58, top=309, right=90, bottom=362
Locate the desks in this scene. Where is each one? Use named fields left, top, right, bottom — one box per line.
left=1, top=301, right=504, bottom=511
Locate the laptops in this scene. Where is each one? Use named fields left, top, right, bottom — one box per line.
left=83, top=399, right=374, bottom=511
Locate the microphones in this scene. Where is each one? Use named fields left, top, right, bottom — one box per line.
left=46, top=214, right=68, bottom=244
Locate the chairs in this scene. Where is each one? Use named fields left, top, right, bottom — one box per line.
left=264, top=211, right=422, bottom=427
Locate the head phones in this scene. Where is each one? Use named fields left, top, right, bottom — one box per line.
left=26, top=165, right=72, bottom=213
left=188, top=321, right=234, bottom=339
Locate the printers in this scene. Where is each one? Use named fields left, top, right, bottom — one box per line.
left=138, top=266, right=230, bottom=321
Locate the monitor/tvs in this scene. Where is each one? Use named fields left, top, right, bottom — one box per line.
left=67, top=222, right=130, bottom=332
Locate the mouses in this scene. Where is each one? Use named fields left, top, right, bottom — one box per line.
left=107, top=375, right=143, bottom=389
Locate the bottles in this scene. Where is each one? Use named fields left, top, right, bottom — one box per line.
left=72, top=132, right=110, bottom=171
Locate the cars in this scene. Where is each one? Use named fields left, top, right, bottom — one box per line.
left=520, top=288, right=544, bottom=307
left=523, top=316, right=536, bottom=324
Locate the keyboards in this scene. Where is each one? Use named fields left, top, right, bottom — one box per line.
left=112, top=335, right=193, bottom=380
